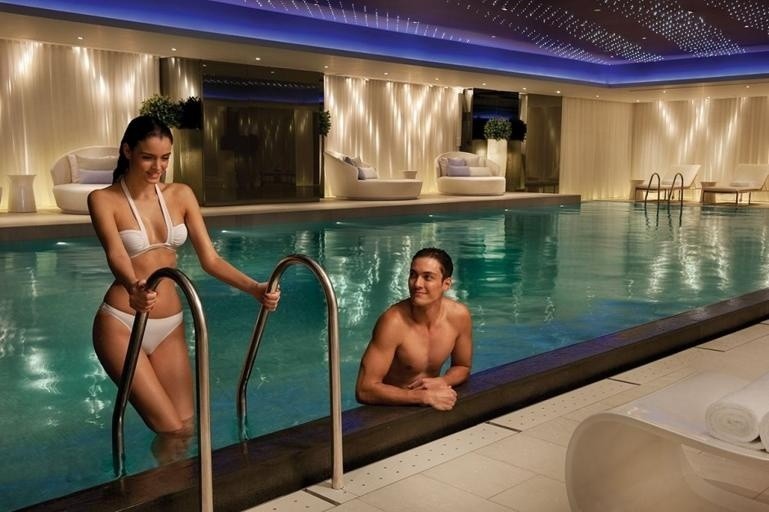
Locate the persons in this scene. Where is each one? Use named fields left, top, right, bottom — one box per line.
left=356, top=248, right=472, bottom=411
left=87, top=116, right=282, bottom=469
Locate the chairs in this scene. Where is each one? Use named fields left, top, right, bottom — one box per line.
left=635, top=165, right=702, bottom=201
left=434, top=152, right=506, bottom=195
left=703, top=164, right=769, bottom=207
left=323, top=150, right=423, bottom=200
left=50, top=145, right=119, bottom=215
left=565, top=371, right=769, bottom=512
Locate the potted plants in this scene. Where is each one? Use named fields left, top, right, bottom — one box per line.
left=484, top=116, right=527, bottom=188
left=139, top=93, right=204, bottom=206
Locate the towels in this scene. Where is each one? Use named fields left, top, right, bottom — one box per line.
left=705, top=370, right=769, bottom=453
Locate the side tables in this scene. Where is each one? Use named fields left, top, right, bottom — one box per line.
left=395, top=170, right=418, bottom=179
left=628, top=178, right=647, bottom=200
left=698, top=181, right=719, bottom=203
left=5, top=174, right=38, bottom=213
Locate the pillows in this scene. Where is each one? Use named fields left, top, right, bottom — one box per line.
left=439, top=154, right=492, bottom=176
left=67, top=154, right=119, bottom=184
left=341, top=152, right=377, bottom=180
